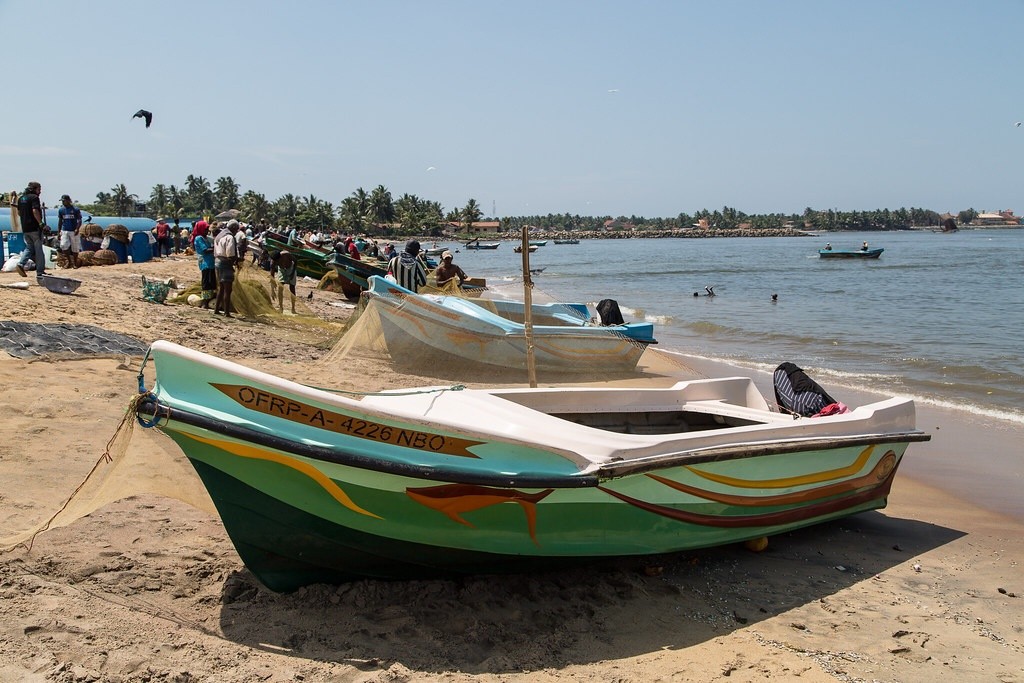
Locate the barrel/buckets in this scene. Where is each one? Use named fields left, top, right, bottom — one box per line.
left=384, top=272, right=396, bottom=284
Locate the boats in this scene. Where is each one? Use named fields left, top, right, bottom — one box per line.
left=466, top=243, right=500, bottom=249
left=361, top=275, right=658, bottom=380
left=513, top=245, right=539, bottom=253
left=932, top=229, right=956, bottom=234
left=818, top=248, right=885, bottom=259
left=554, top=239, right=580, bottom=244
left=248, top=229, right=488, bottom=302
left=134, top=338, right=931, bottom=596
left=529, top=241, right=548, bottom=246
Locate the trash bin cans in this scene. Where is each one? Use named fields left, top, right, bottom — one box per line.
left=129, top=230, right=153, bottom=264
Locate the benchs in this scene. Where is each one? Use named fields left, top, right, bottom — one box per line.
left=552, top=313, right=589, bottom=326
left=684, top=400, right=808, bottom=424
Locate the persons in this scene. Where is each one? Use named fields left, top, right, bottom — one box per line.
left=255, top=217, right=327, bottom=314
left=327, top=228, right=469, bottom=303
left=156, top=217, right=171, bottom=257
left=861, top=241, right=868, bottom=251
left=826, top=243, right=832, bottom=251
left=171, top=219, right=183, bottom=254
left=57, top=193, right=83, bottom=268
left=12, top=182, right=54, bottom=278
left=181, top=219, right=255, bottom=318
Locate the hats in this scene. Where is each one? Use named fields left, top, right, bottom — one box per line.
left=441, top=250, right=451, bottom=260
left=345, top=237, right=352, bottom=242
left=59, top=195, right=69, bottom=202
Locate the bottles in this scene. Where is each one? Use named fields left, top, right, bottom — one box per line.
left=128, top=255, right=132, bottom=265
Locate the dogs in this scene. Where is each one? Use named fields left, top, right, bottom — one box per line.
left=184, top=246, right=194, bottom=255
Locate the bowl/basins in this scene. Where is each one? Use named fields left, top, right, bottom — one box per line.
left=42, top=274, right=82, bottom=294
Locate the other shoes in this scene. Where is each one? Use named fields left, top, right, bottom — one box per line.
left=17, top=264, right=27, bottom=277
left=36, top=272, right=52, bottom=278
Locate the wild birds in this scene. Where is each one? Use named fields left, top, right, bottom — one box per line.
left=130, top=109, right=152, bottom=128
left=84, top=215, right=92, bottom=223
left=307, top=291, right=313, bottom=300
left=770, top=294, right=777, bottom=301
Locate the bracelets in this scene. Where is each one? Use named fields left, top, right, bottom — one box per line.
left=39, top=223, right=46, bottom=228
